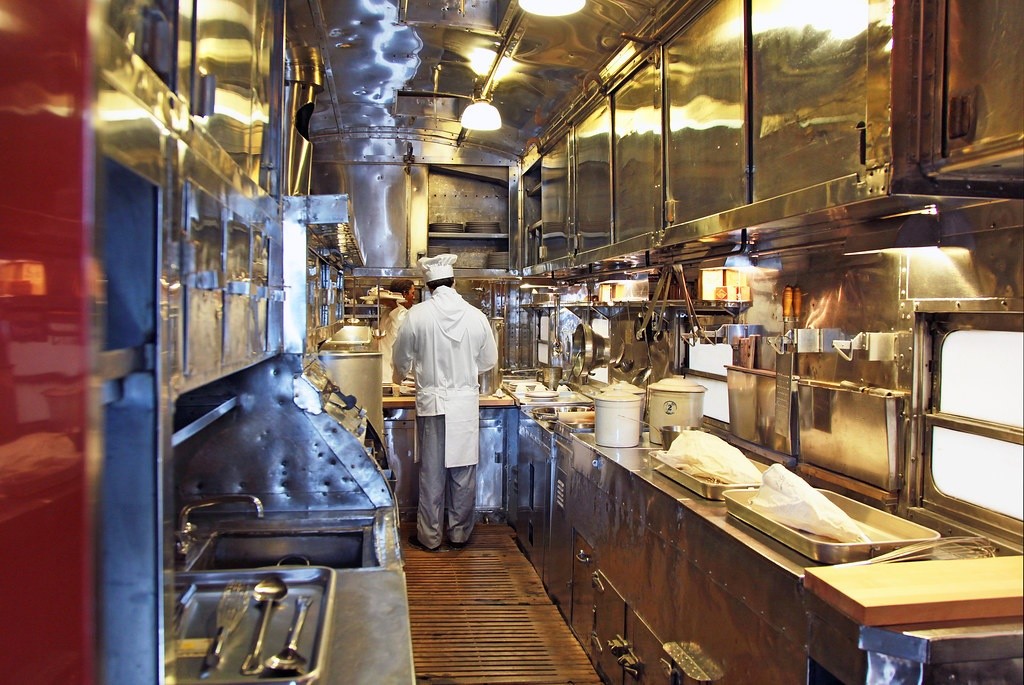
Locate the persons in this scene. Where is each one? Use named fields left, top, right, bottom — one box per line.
left=373, top=297, right=406, bottom=329
left=392, top=254, right=498, bottom=553
left=377, top=276, right=416, bottom=385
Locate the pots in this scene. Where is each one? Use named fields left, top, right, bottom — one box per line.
left=479, top=316, right=504, bottom=395
left=541, top=367, right=562, bottom=392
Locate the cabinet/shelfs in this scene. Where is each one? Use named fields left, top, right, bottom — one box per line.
left=428, top=230, right=521, bottom=282
left=343, top=303, right=382, bottom=319
left=514, top=0, right=1024, bottom=273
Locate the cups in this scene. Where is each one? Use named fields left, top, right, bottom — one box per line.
left=601, top=380, right=645, bottom=436
left=593, top=390, right=643, bottom=448
left=647, top=375, right=706, bottom=444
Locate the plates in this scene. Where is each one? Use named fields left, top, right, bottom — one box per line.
left=526, top=391, right=559, bottom=402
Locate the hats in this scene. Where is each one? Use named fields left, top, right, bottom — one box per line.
left=417, top=253, right=458, bottom=283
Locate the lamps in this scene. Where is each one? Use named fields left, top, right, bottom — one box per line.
left=460, top=88, right=501, bottom=130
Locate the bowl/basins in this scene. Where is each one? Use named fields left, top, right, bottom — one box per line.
left=659, top=423, right=710, bottom=451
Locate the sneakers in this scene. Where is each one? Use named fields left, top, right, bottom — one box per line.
left=408, top=535, right=440, bottom=553
left=447, top=539, right=468, bottom=550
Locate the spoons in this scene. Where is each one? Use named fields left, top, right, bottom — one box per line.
left=264, top=594, right=315, bottom=674
left=241, top=579, right=287, bottom=676
